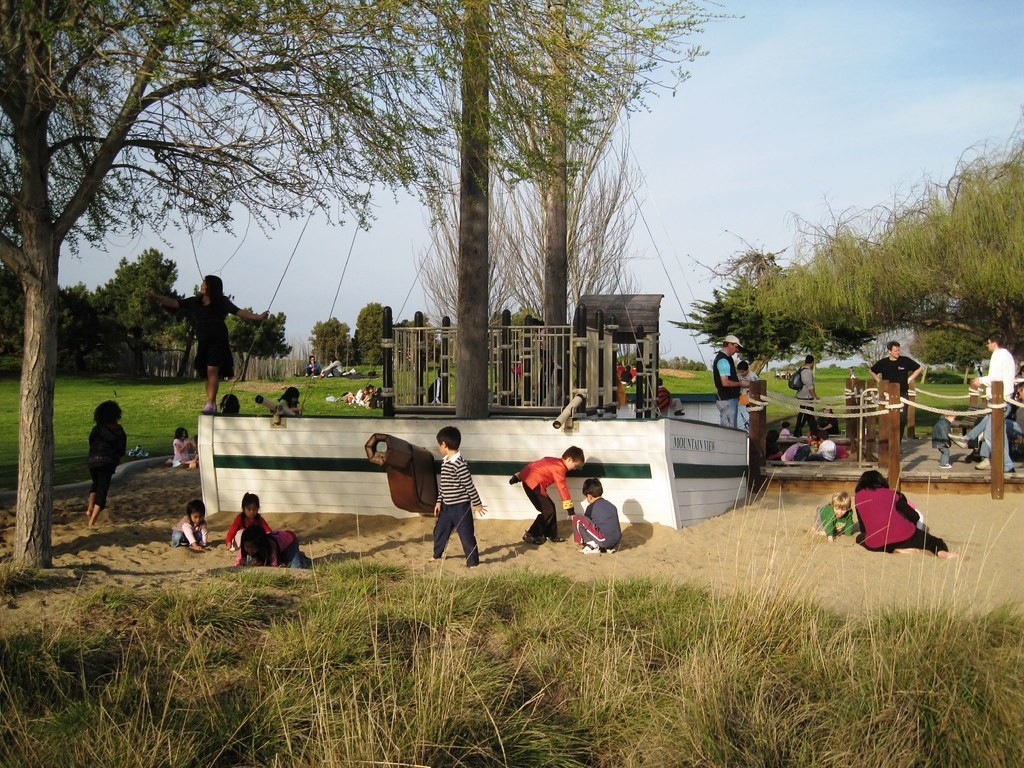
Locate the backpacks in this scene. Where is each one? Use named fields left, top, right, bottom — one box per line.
left=788, top=366, right=809, bottom=391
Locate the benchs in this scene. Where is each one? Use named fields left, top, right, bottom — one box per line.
left=950, top=403, right=990, bottom=434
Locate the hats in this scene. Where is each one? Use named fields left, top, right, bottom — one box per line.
left=724, top=334, right=743, bottom=348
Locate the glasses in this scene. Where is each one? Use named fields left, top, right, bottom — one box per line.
left=987, top=341, right=991, bottom=345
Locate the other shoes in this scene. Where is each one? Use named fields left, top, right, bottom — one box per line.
left=948, top=433, right=968, bottom=449
left=201, top=404, right=217, bottom=413
left=940, top=463, right=952, bottom=469
left=674, top=411, right=685, bottom=416
left=1005, top=467, right=1015, bottom=473
left=522, top=530, right=541, bottom=545
left=975, top=458, right=991, bottom=469
left=578, top=543, right=601, bottom=555
left=600, top=547, right=616, bottom=554
left=545, top=536, right=565, bottom=542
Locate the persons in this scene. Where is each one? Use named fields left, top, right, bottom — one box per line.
left=171, top=499, right=208, bottom=552
left=854, top=470, right=959, bottom=560
left=141, top=274, right=270, bottom=414
left=427, top=328, right=568, bottom=408
left=572, top=478, right=622, bottom=555
left=775, top=367, right=793, bottom=379
left=850, top=367, right=856, bottom=379
left=509, top=446, right=584, bottom=545
left=868, top=341, right=922, bottom=444
left=713, top=335, right=761, bottom=429
left=226, top=492, right=312, bottom=569
left=340, top=384, right=382, bottom=408
left=304, top=355, right=344, bottom=379
left=614, top=361, right=685, bottom=418
left=765, top=403, right=841, bottom=467
left=812, top=491, right=855, bottom=544
left=219, top=387, right=305, bottom=418
left=932, top=328, right=1024, bottom=474
left=793, top=355, right=821, bottom=440
left=427, top=425, right=488, bottom=570
left=172, top=387, right=200, bottom=467
left=86, top=400, right=127, bottom=530
